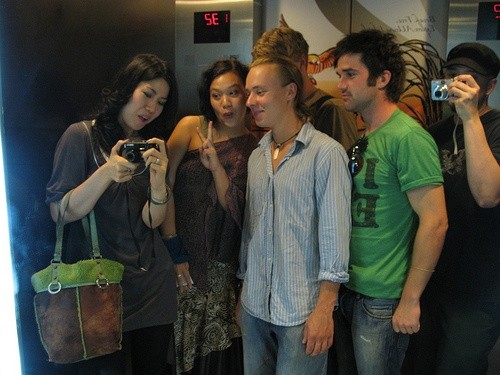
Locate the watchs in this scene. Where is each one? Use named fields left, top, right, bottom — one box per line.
left=237, top=282, right=243, bottom=289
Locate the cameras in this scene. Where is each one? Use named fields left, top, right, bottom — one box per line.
left=431, top=79, right=454, bottom=101
left=118, top=142, right=159, bottom=162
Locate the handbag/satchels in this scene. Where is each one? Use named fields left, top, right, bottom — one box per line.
left=29, top=189, right=125, bottom=364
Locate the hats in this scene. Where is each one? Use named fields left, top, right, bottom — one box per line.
left=442, top=43, right=500, bottom=80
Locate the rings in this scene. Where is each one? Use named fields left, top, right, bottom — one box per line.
left=127, top=168, right=131, bottom=174
left=156, top=158, right=161, bottom=164
left=176, top=274, right=182, bottom=278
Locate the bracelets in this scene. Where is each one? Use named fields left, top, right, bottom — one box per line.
left=147, top=183, right=172, bottom=205
left=159, top=232, right=180, bottom=241
left=410, top=261, right=437, bottom=274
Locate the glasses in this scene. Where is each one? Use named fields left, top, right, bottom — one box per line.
left=347, top=141, right=363, bottom=176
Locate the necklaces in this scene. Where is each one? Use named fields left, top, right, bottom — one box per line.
left=272, top=130, right=299, bottom=160
left=125, top=131, right=140, bottom=138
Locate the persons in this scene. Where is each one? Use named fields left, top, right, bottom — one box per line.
left=164, top=60, right=261, bottom=375
left=249, top=27, right=358, bottom=157
left=415, top=41, right=500, bottom=375
left=232, top=55, right=354, bottom=374
left=45, top=53, right=180, bottom=375
left=329, top=29, right=450, bottom=375
